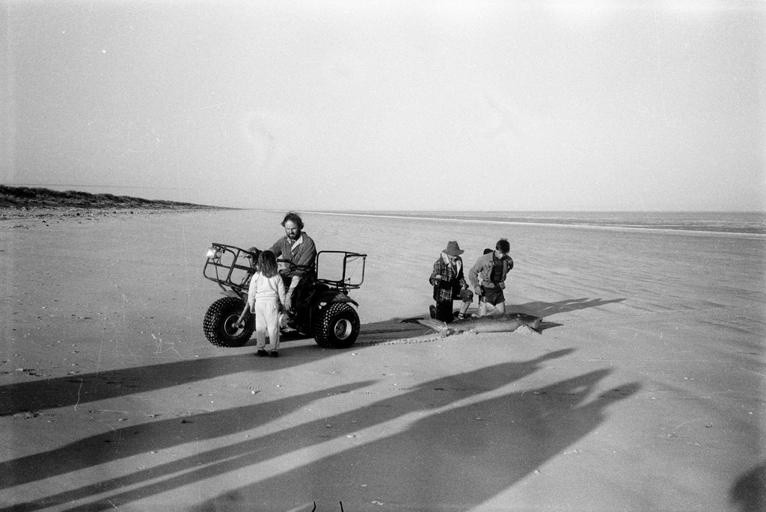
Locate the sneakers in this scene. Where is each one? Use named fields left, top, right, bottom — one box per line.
left=429, top=304, right=436, bottom=318
left=270, top=350, right=279, bottom=358
left=256, top=350, right=268, bottom=357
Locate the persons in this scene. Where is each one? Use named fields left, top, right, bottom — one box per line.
left=429, top=240, right=473, bottom=322
left=248, top=250, right=286, bottom=358
left=467, top=239, right=514, bottom=317
left=238, top=213, right=317, bottom=336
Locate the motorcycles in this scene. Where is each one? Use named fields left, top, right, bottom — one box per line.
left=202, top=243, right=367, bottom=349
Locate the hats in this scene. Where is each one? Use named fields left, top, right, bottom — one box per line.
left=441, top=240, right=465, bottom=257
